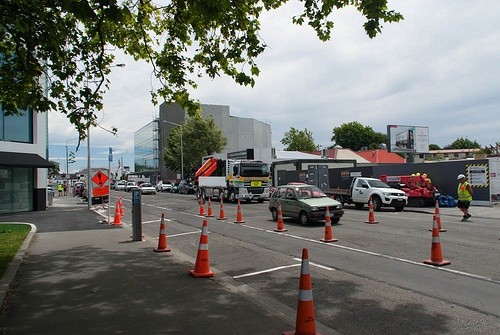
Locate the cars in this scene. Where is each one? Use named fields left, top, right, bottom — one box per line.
left=156, top=180, right=198, bottom=194
left=74, top=181, right=87, bottom=197
left=114, top=180, right=157, bottom=195
left=268, top=184, right=344, bottom=226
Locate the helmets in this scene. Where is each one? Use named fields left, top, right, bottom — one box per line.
left=457, top=174, right=465, bottom=179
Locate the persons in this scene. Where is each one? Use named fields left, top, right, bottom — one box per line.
left=57, top=184, right=63, bottom=197
left=63, top=181, right=68, bottom=196
left=456, top=174, right=473, bottom=221
left=422, top=173, right=431, bottom=185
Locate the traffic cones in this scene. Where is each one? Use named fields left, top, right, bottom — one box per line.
left=428, top=200, right=447, bottom=232
left=234, top=199, right=245, bottom=223
left=189, top=220, right=214, bottom=278
left=217, top=197, right=227, bottom=220
left=364, top=196, right=379, bottom=224
left=153, top=212, right=171, bottom=252
left=273, top=202, right=288, bottom=232
left=111, top=194, right=124, bottom=225
left=320, top=206, right=338, bottom=242
left=198, top=196, right=206, bottom=215
left=205, top=197, right=214, bottom=217
left=423, top=215, right=451, bottom=266
left=282, top=247, right=320, bottom=335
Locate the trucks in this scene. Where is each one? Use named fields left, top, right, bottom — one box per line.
left=83, top=170, right=111, bottom=204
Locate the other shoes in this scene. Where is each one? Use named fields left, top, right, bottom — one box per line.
left=461, top=216, right=467, bottom=221
left=467, top=213, right=472, bottom=219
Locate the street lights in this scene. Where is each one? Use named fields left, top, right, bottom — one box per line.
left=86, top=63, right=126, bottom=210
left=152, top=119, right=184, bottom=180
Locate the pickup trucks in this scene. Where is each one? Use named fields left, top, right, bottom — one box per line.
left=326, top=176, right=409, bottom=212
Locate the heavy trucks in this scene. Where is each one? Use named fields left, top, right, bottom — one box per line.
left=196, top=157, right=274, bottom=203
